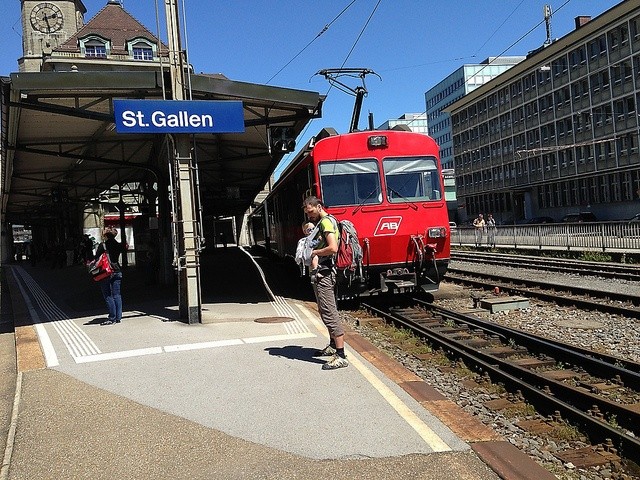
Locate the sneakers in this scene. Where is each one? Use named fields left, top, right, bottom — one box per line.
left=100, top=319, right=115, bottom=325
left=116, top=318, right=121, bottom=323
left=316, top=345, right=338, bottom=356
left=322, top=355, right=349, bottom=369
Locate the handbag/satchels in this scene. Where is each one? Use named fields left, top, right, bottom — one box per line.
left=85, top=251, right=117, bottom=286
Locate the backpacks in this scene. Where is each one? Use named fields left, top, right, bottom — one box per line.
left=326, top=215, right=363, bottom=279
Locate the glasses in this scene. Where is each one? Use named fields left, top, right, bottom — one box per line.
left=307, top=227, right=315, bottom=232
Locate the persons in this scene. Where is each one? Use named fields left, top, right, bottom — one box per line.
left=296, top=221, right=324, bottom=282
left=95, top=226, right=123, bottom=325
left=64, top=235, right=75, bottom=268
left=486, top=213, right=495, bottom=245
left=83, top=234, right=93, bottom=265
left=472, top=213, right=485, bottom=246
left=302, top=196, right=349, bottom=370
left=16, top=239, right=60, bottom=267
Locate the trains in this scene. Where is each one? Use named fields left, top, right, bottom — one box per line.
left=247, top=112, right=450, bottom=303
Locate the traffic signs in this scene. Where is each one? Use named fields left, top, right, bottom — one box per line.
left=113, top=99, right=244, bottom=133
